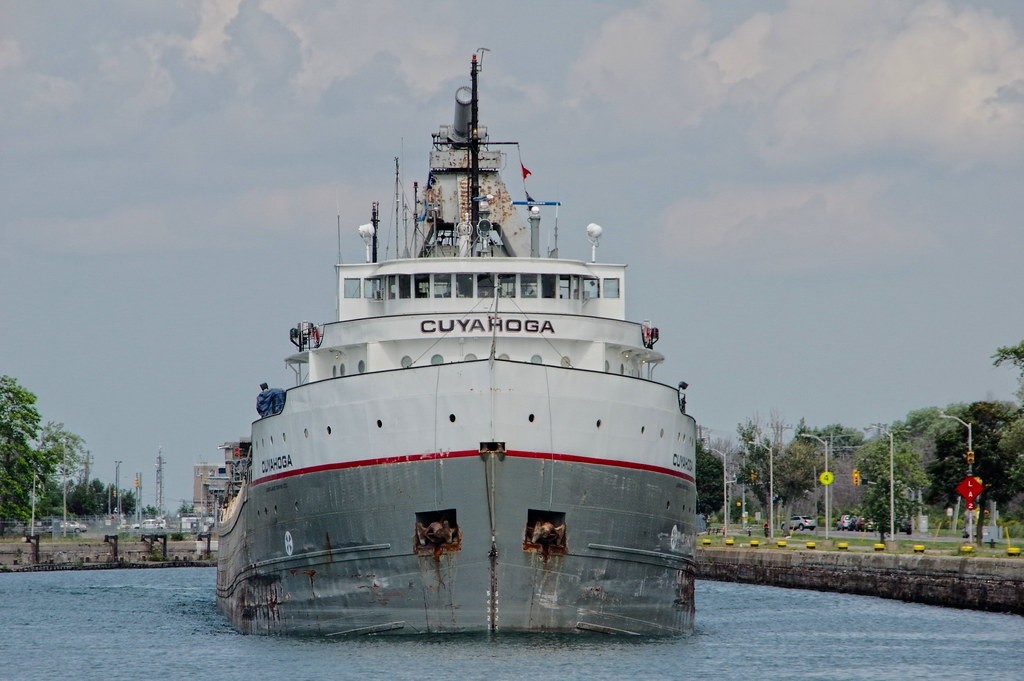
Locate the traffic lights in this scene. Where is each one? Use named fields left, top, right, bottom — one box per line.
left=853, top=470, right=859, bottom=486
left=967, top=452, right=974, bottom=464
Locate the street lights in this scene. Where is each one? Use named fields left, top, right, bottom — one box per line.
left=801, top=434, right=829, bottom=539
left=748, top=441, right=773, bottom=539
left=938, top=414, right=973, bottom=543
left=872, top=426, right=895, bottom=542
left=705, top=448, right=727, bottom=537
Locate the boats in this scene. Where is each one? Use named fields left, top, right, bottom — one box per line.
left=216, top=48, right=700, bottom=641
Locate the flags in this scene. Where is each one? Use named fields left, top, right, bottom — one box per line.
left=522, top=165, right=530, bottom=178
left=528, top=193, right=535, bottom=212
left=428, top=172, right=439, bottom=188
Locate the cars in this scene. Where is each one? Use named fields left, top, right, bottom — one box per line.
left=49, top=521, right=87, bottom=533
left=889, top=516, right=912, bottom=535
left=781, top=515, right=817, bottom=531
left=855, top=516, right=873, bottom=532
left=131, top=519, right=166, bottom=530
left=837, top=515, right=857, bottom=531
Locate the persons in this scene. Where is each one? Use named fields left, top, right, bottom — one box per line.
left=748, top=523, right=753, bottom=535
left=763, top=523, right=769, bottom=536
